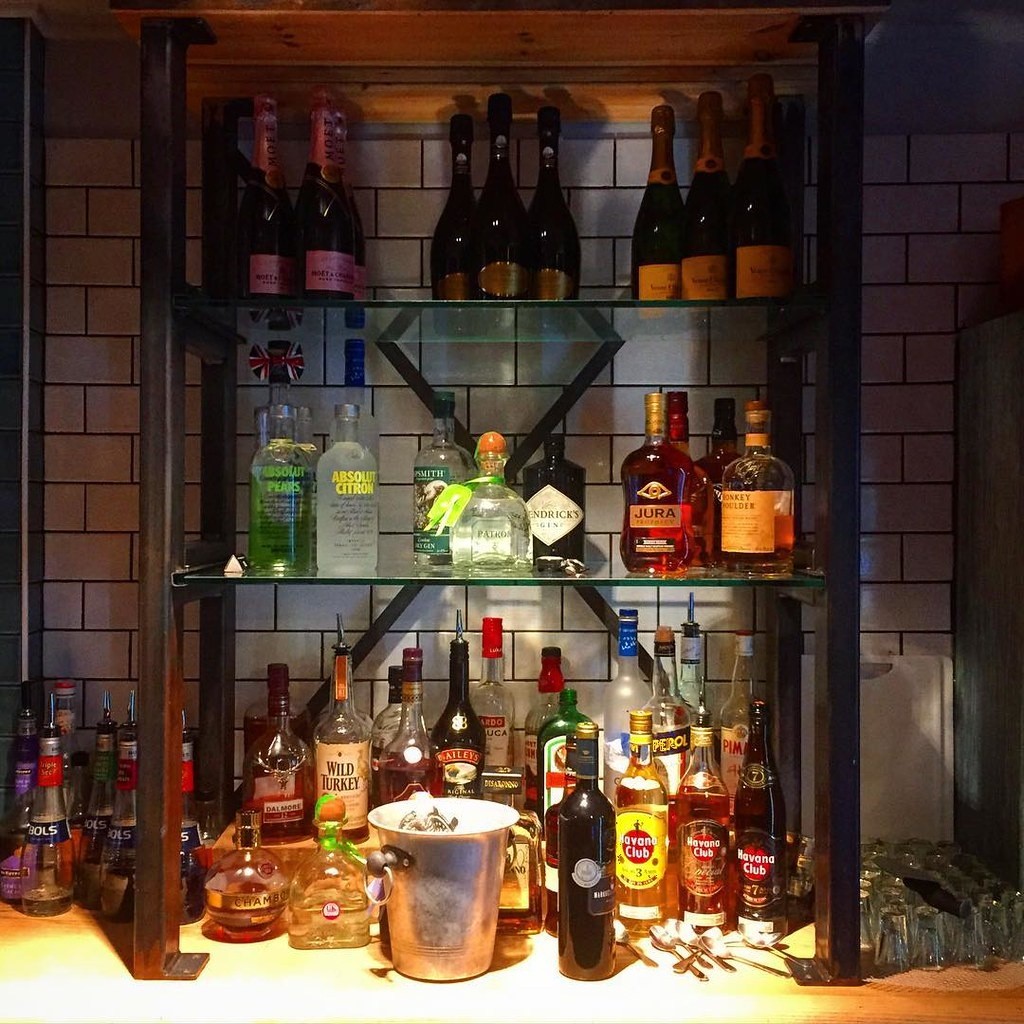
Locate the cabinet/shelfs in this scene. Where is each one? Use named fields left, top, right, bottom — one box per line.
left=111, top=0, right=861, bottom=991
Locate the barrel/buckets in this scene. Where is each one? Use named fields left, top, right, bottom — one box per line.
left=364, top=797, right=520, bottom=981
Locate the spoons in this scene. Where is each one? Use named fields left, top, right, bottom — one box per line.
left=611, top=918, right=656, bottom=969
left=649, top=919, right=813, bottom=982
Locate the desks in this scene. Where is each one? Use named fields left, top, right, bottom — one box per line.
left=0, top=844, right=1024, bottom=1024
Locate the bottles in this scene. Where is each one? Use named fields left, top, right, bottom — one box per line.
left=414, top=390, right=588, bottom=575
left=238, top=84, right=366, bottom=301
left=630, top=73, right=795, bottom=298
left=248, top=401, right=378, bottom=573
left=430, top=92, right=581, bottom=302
left=620, top=391, right=796, bottom=578
left=204, top=592, right=791, bottom=983
left=1, top=679, right=207, bottom=925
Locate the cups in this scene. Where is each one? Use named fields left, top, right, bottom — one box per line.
left=858, top=836, right=1023, bottom=975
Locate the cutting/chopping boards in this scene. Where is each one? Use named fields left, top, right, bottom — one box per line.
left=800, top=654, right=955, bottom=846
